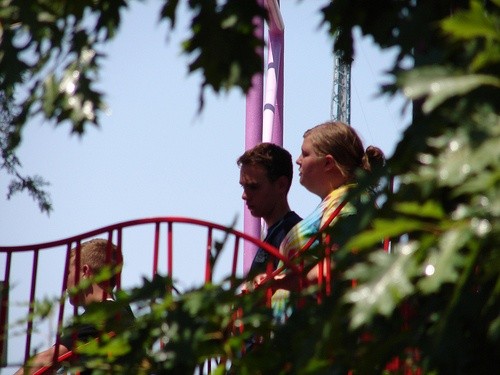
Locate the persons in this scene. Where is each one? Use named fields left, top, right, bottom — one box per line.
left=237, top=142, right=306, bottom=359
left=271, top=119, right=390, bottom=336
left=14, top=236, right=140, bottom=374
